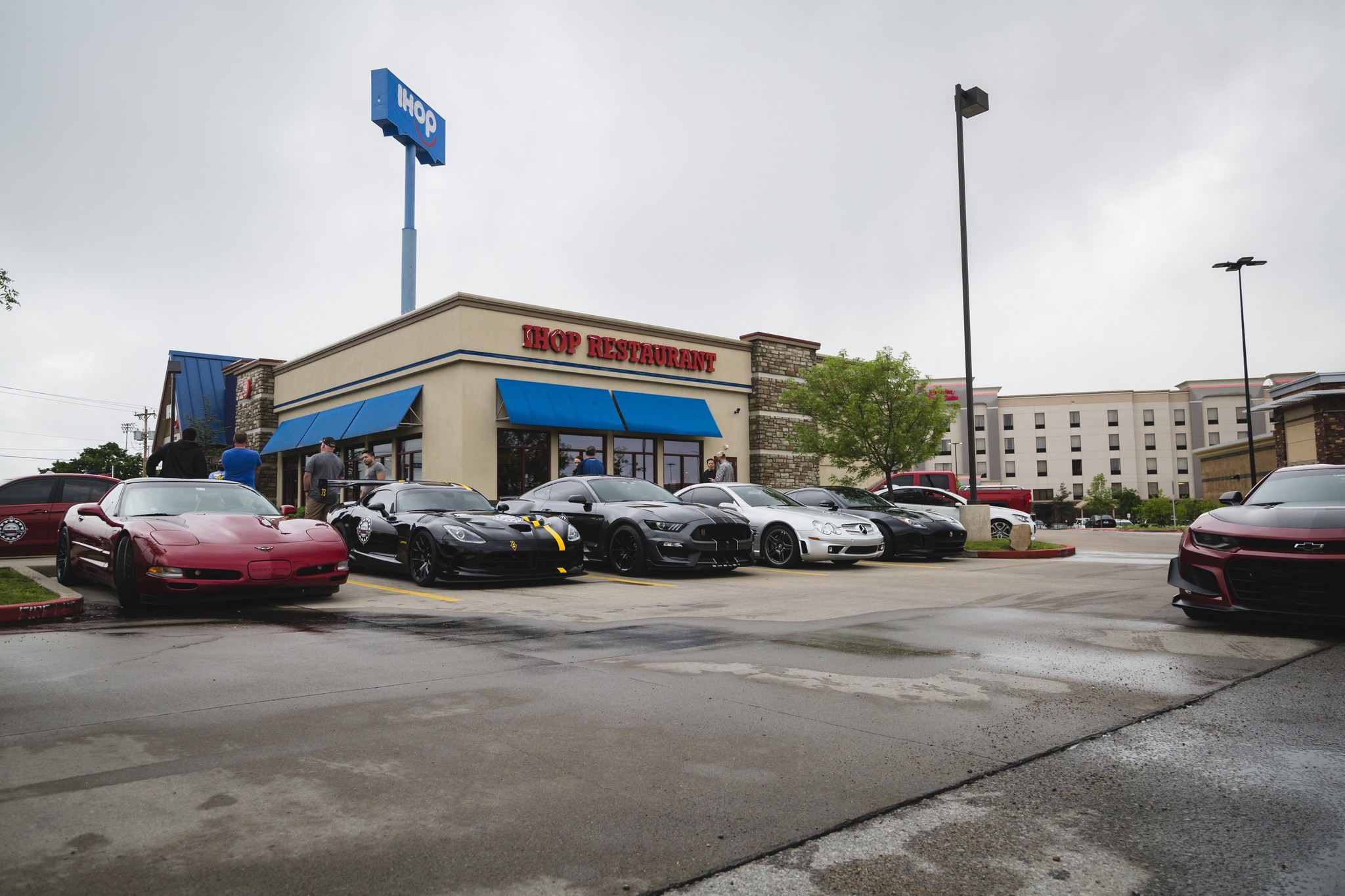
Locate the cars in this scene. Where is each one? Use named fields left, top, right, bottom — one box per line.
left=1049, top=523, right=1071, bottom=529
left=872, top=484, right=1037, bottom=541
left=1034, top=520, right=1048, bottom=529
left=0, top=473, right=124, bottom=557
left=1084, top=514, right=1117, bottom=529
left=1114, top=519, right=1134, bottom=528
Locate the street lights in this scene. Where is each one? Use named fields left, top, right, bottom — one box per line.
left=948, top=442, right=963, bottom=482
left=1211, top=256, right=1268, bottom=486
left=955, top=83, right=991, bottom=501
left=121, top=423, right=137, bottom=453
left=665, top=462, right=676, bottom=488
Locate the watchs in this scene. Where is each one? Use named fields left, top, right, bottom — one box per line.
left=304, top=489, right=309, bottom=493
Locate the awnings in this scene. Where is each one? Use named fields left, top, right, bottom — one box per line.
left=259, top=385, right=423, bottom=455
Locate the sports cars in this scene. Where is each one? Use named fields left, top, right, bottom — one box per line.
left=671, top=482, right=885, bottom=569
left=495, top=474, right=757, bottom=577
left=1167, top=463, right=1345, bottom=630
left=782, top=485, right=969, bottom=562
left=56, top=477, right=351, bottom=613
left=317, top=478, right=589, bottom=589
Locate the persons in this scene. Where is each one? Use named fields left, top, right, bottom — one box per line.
left=221, top=431, right=263, bottom=491
left=360, top=449, right=386, bottom=500
left=304, top=436, right=344, bottom=522
left=708, top=451, right=734, bottom=483
left=699, top=458, right=717, bottom=483
left=146, top=427, right=209, bottom=479
left=572, top=446, right=604, bottom=476
left=208, top=459, right=225, bottom=479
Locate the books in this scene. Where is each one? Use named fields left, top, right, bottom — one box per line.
left=495, top=378, right=723, bottom=438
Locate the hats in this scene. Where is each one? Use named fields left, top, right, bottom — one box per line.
left=715, top=451, right=725, bottom=459
left=319, top=437, right=337, bottom=448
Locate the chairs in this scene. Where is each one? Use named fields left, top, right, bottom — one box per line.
left=195, top=497, right=224, bottom=512
left=926, top=492, right=935, bottom=505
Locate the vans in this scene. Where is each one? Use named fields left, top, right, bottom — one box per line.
left=1073, top=518, right=1091, bottom=529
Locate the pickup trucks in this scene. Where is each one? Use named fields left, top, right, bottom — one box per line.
left=863, top=470, right=1037, bottom=522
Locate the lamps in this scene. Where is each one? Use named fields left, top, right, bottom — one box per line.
left=734, top=408, right=740, bottom=414
left=1230, top=475, right=1239, bottom=479
